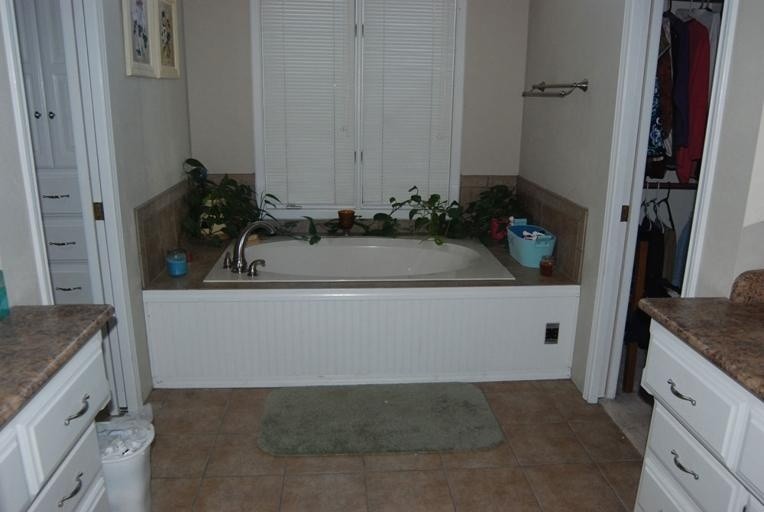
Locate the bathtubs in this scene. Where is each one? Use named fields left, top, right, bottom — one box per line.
left=201, top=233, right=516, bottom=282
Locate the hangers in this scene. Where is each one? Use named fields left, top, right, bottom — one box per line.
left=641, top=180, right=676, bottom=235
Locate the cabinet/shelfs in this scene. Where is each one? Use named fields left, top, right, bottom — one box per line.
left=0, top=330, right=116, bottom=512
left=11, top=0, right=98, bottom=305
left=632, top=319, right=764, bottom=512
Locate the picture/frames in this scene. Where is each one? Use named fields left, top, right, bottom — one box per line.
left=123, top=0, right=182, bottom=80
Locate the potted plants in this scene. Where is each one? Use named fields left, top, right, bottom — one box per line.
left=338, top=209, right=354, bottom=227
left=466, top=184, right=516, bottom=239
left=183, top=159, right=256, bottom=241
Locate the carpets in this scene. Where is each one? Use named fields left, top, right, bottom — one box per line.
left=255, top=381, right=503, bottom=456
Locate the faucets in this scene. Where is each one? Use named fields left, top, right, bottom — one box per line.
left=230, top=219, right=278, bottom=274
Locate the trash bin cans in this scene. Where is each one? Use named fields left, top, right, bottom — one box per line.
left=96, top=420, right=156, bottom=512
